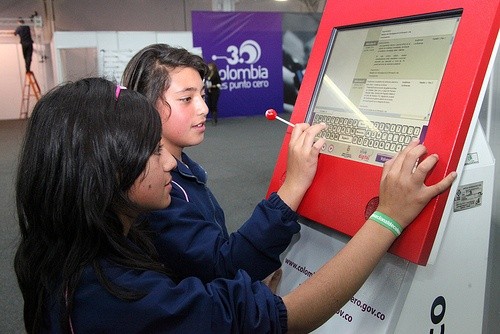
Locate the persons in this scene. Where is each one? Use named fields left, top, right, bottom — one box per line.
left=14, top=75, right=458, bottom=334
left=13, top=16, right=36, bottom=74
left=122, top=42, right=330, bottom=284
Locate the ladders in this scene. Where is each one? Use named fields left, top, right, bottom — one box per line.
left=19, top=69, right=43, bottom=120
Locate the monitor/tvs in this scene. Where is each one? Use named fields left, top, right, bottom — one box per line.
left=305, top=8, right=462, bottom=174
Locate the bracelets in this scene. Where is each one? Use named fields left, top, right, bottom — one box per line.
left=368, top=210, right=404, bottom=237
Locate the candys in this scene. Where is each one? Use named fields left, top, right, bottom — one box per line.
left=265, top=108, right=295, bottom=127
